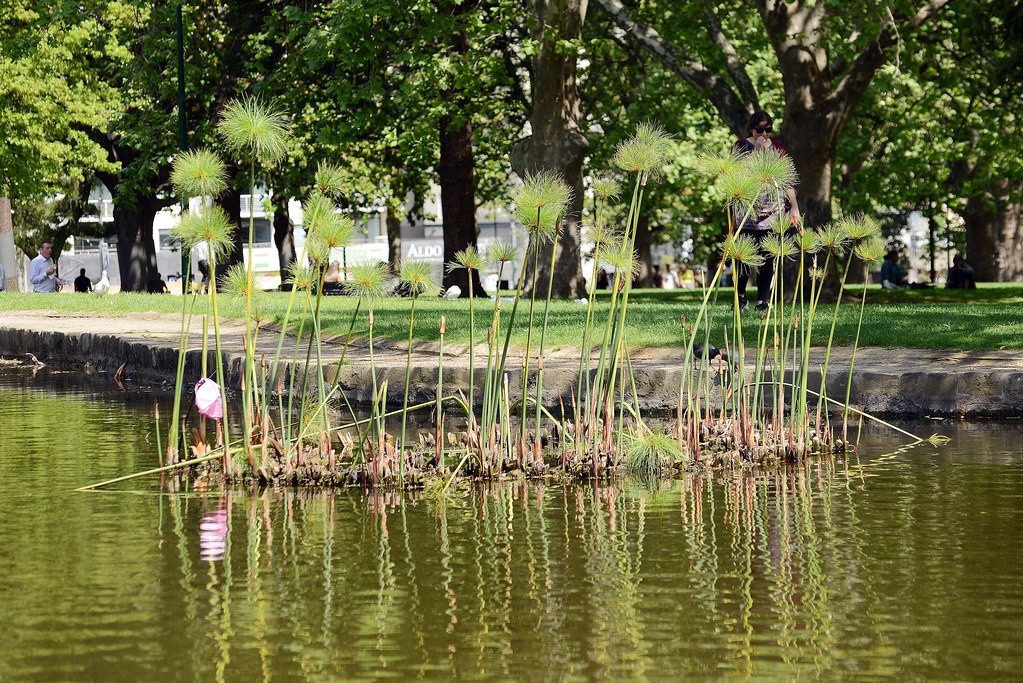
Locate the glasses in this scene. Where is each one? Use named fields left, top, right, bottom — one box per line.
left=753, top=127, right=773, bottom=134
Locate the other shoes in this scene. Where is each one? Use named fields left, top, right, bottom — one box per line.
left=754, top=302, right=768, bottom=312
left=730, top=295, right=749, bottom=310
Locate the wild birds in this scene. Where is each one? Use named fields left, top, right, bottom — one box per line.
left=692, top=341, right=741, bottom=371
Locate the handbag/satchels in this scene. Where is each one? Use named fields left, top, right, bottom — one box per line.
left=738, top=210, right=776, bottom=227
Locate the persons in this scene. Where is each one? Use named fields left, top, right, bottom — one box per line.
left=74, top=268, right=93, bottom=293
left=730, top=109, right=799, bottom=312
left=158, top=272, right=167, bottom=293
left=576, top=277, right=589, bottom=299
left=945, top=254, right=975, bottom=290
left=596, top=258, right=735, bottom=289
left=324, top=260, right=340, bottom=281
left=880, top=249, right=907, bottom=289
left=30, top=240, right=63, bottom=293
left=0, top=264, right=7, bottom=292
